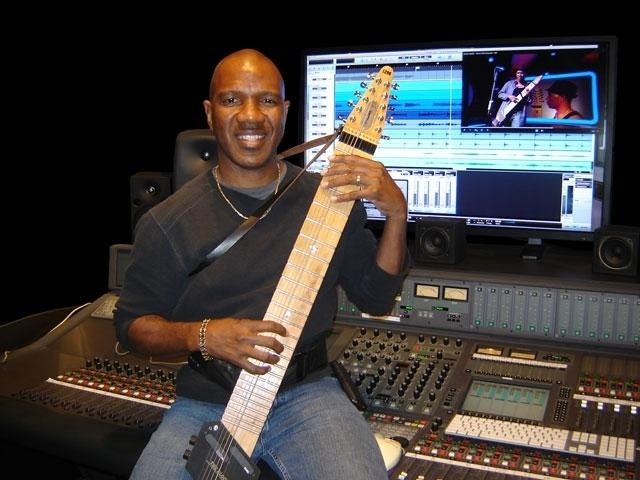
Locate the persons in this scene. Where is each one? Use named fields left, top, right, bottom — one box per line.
left=489, top=66, right=533, bottom=129
left=544, top=79, right=585, bottom=121
left=109, top=47, right=409, bottom=480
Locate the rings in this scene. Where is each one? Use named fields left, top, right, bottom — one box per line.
left=355, top=171, right=363, bottom=186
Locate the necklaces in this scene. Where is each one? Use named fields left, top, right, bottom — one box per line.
left=212, top=161, right=282, bottom=224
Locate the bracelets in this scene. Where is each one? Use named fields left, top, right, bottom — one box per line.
left=199, top=317, right=215, bottom=363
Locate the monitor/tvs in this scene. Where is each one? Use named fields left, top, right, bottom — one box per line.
left=299, top=34, right=619, bottom=244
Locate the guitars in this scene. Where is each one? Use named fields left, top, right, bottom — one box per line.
left=182, top=65, right=399, bottom=479
left=491, top=75, right=543, bottom=127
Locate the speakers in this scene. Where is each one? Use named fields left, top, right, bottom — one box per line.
left=174, top=129, right=218, bottom=193
left=130, top=171, right=172, bottom=242
left=414, top=216, right=466, bottom=264
left=591, top=224, right=640, bottom=283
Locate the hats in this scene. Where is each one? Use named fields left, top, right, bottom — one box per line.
left=544, top=79, right=579, bottom=98
left=517, top=64, right=525, bottom=71
